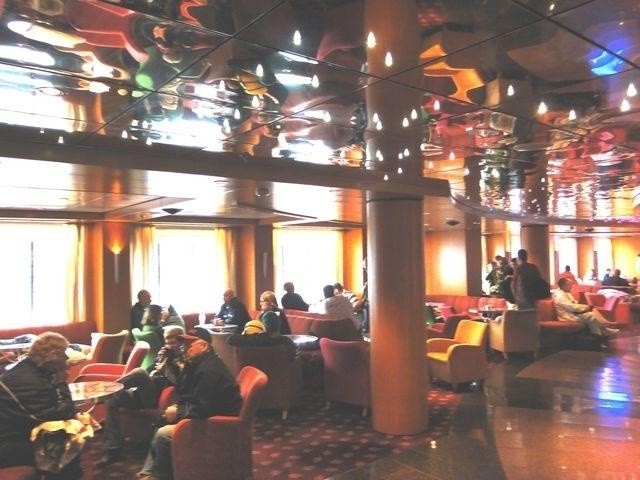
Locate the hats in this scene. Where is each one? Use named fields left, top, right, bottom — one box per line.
left=179, top=326, right=213, bottom=345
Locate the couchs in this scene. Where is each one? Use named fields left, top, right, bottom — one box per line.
left=550, top=285, right=596, bottom=302
left=248, top=310, right=354, bottom=340
left=181, top=313, right=199, bottom=336
left=536, top=300, right=586, bottom=332
left=205, top=312, right=217, bottom=323
left=0, top=321, right=97, bottom=345
left=425, top=295, right=507, bottom=320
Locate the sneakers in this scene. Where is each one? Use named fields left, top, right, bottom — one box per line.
left=603, top=327, right=621, bottom=336
left=600, top=343, right=607, bottom=350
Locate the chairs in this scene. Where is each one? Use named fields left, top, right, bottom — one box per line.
left=603, top=286, right=633, bottom=295
left=628, top=302, right=640, bottom=312
left=116, top=386, right=176, bottom=428
left=595, top=297, right=619, bottom=312
left=72, top=340, right=151, bottom=382
left=584, top=292, right=606, bottom=306
left=226, top=335, right=296, bottom=414
left=65, top=329, right=129, bottom=382
left=426, top=320, right=490, bottom=391
left=490, top=309, right=538, bottom=359
left=132, top=327, right=162, bottom=373
left=0, top=466, right=36, bottom=480
left=172, top=366, right=268, bottom=480
left=427, top=315, right=471, bottom=338
left=320, top=338, right=370, bottom=416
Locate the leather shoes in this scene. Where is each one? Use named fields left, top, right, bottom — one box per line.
left=92, top=450, right=126, bottom=469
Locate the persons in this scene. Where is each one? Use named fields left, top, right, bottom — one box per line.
left=604, top=269, right=610, bottom=281
left=135, top=328, right=243, bottom=480
left=511, top=250, right=542, bottom=351
left=310, top=285, right=362, bottom=337
left=225, top=312, right=294, bottom=363
left=0, top=334, right=91, bottom=363
left=0, top=332, right=84, bottom=480
left=330, top=283, right=359, bottom=313
left=486, top=256, right=513, bottom=300
left=215, top=290, right=252, bottom=330
left=554, top=278, right=620, bottom=348
left=281, top=283, right=308, bottom=312
left=603, top=270, right=629, bottom=287
left=258, top=291, right=291, bottom=334
left=566, top=279, right=620, bottom=336
left=91, top=326, right=186, bottom=467
left=131, top=290, right=151, bottom=340
left=561, top=266, right=577, bottom=285
left=360, top=281, right=368, bottom=331
left=141, top=304, right=165, bottom=345
